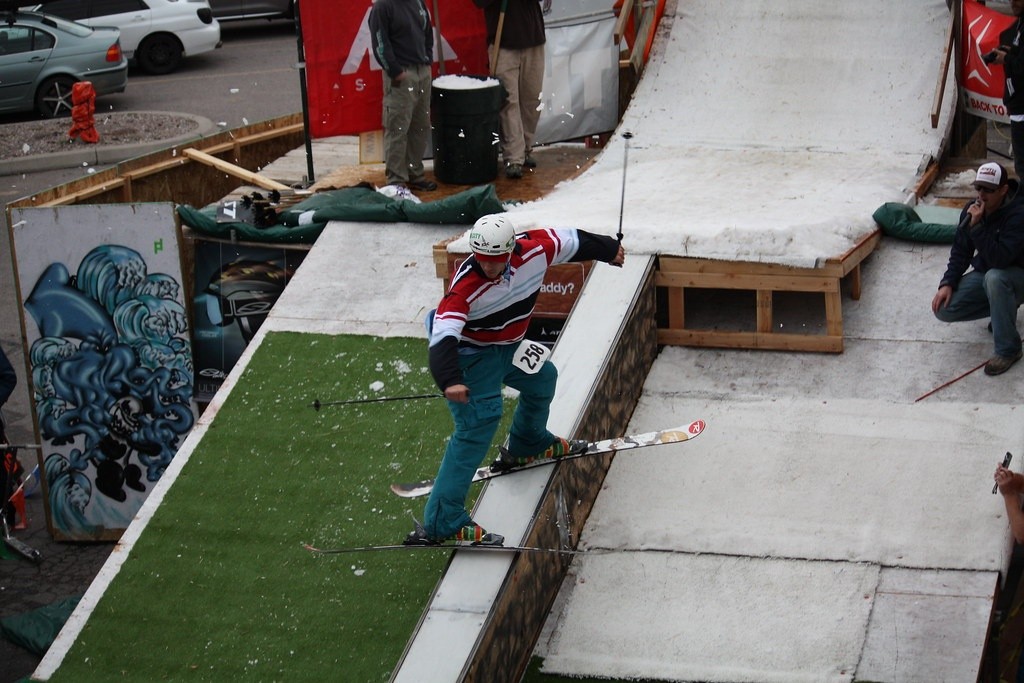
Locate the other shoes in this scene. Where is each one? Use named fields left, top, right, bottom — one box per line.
left=984, top=351, right=1022, bottom=376
left=525, top=155, right=537, bottom=168
left=409, top=179, right=438, bottom=192
left=506, top=164, right=522, bottom=178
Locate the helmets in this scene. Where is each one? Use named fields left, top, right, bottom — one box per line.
left=468, top=214, right=517, bottom=265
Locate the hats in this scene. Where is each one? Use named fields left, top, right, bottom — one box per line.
left=969, top=161, right=1008, bottom=190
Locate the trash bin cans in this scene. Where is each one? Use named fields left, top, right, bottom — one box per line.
left=433, top=75, right=503, bottom=187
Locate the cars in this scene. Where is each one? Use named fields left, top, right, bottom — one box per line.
left=0, top=5, right=130, bottom=119
left=1, top=0, right=223, bottom=77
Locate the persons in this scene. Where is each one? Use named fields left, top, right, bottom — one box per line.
left=473, top=0, right=547, bottom=179
left=992, top=0, right=1024, bottom=181
left=0, top=345, right=18, bottom=534
left=928, top=162, right=1024, bottom=376
left=422, top=214, right=624, bottom=545
left=993, top=462, right=1024, bottom=546
left=367, top=0, right=438, bottom=195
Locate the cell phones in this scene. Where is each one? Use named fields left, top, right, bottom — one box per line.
left=1002, top=452, right=1013, bottom=470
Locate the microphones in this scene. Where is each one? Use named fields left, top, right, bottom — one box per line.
left=959, top=201, right=981, bottom=230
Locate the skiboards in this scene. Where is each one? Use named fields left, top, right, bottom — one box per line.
left=299, top=416, right=709, bottom=557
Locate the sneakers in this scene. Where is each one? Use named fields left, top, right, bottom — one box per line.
left=517, top=436, right=571, bottom=463
left=446, top=520, right=488, bottom=541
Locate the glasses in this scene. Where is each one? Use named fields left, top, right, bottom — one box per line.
left=974, top=185, right=995, bottom=193
